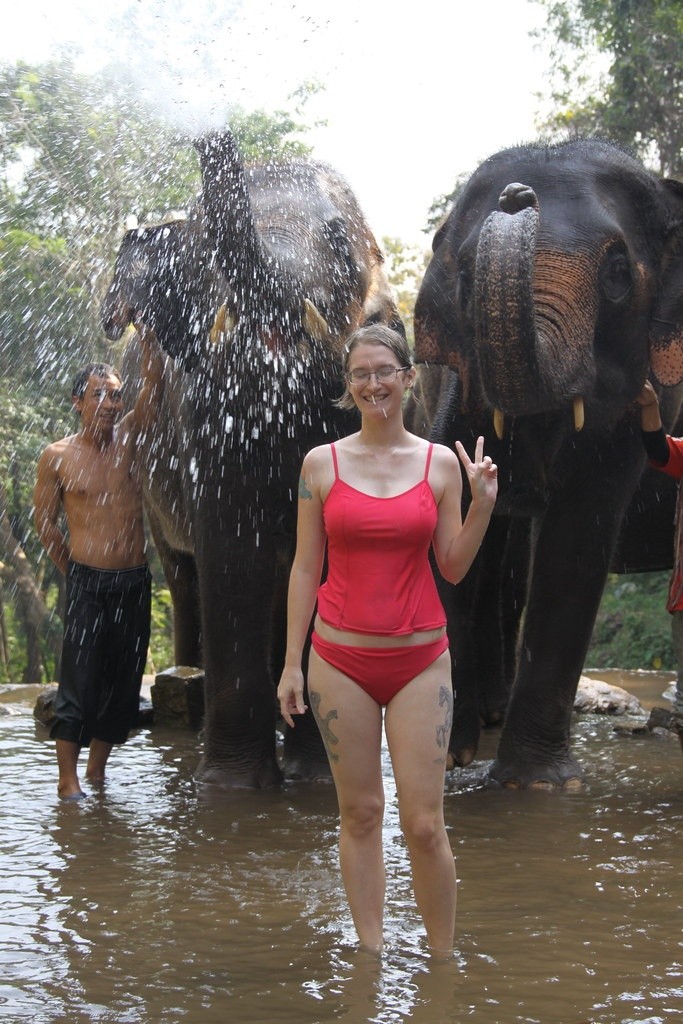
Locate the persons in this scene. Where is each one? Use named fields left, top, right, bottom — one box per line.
left=33, top=311, right=166, bottom=801
left=635, top=372, right=683, bottom=762
left=275, top=323, right=499, bottom=952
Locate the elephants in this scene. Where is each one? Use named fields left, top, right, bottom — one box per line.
left=403, top=137, right=683, bottom=794
left=94, top=124, right=413, bottom=797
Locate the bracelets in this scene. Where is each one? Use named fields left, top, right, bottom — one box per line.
left=640, top=398, right=658, bottom=408
